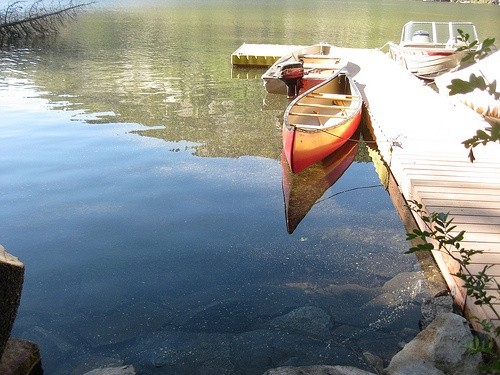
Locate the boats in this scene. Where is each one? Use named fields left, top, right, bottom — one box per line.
left=387, top=20, right=481, bottom=81
left=262, top=92, right=293, bottom=111
left=282, top=65, right=363, bottom=172
left=261, top=44, right=342, bottom=94
left=283, top=127, right=361, bottom=234
